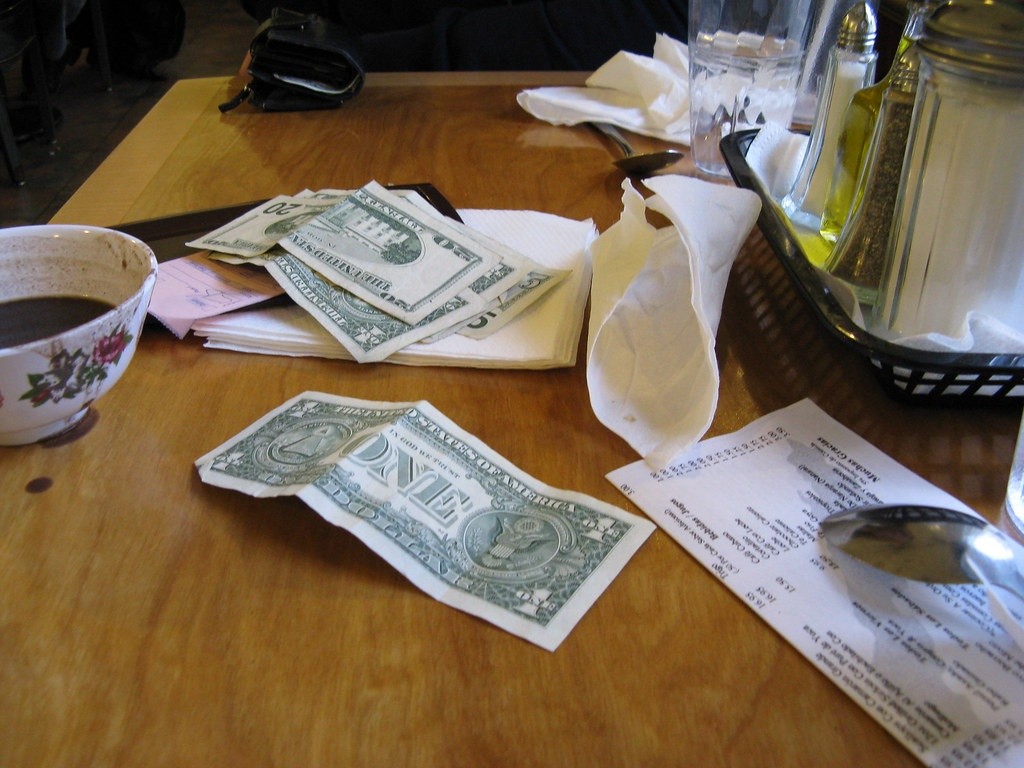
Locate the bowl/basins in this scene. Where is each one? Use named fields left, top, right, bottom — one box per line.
left=0, top=223, right=159, bottom=446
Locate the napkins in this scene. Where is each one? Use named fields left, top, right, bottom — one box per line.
left=201, top=30, right=764, bottom=470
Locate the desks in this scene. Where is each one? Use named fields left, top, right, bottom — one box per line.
left=1, top=70, right=1024, bottom=768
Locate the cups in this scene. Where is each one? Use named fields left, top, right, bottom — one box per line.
left=687, top=0, right=818, bottom=180
left=872, top=0, right=1024, bottom=357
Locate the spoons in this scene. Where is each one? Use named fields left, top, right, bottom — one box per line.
left=822, top=503, right=1024, bottom=602
left=591, top=122, right=685, bottom=173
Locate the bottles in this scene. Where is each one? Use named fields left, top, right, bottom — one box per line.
left=821, top=43, right=922, bottom=307
left=780, top=0, right=879, bottom=233
left=999, top=412, right=1024, bottom=548
left=820, top=0, right=944, bottom=249
